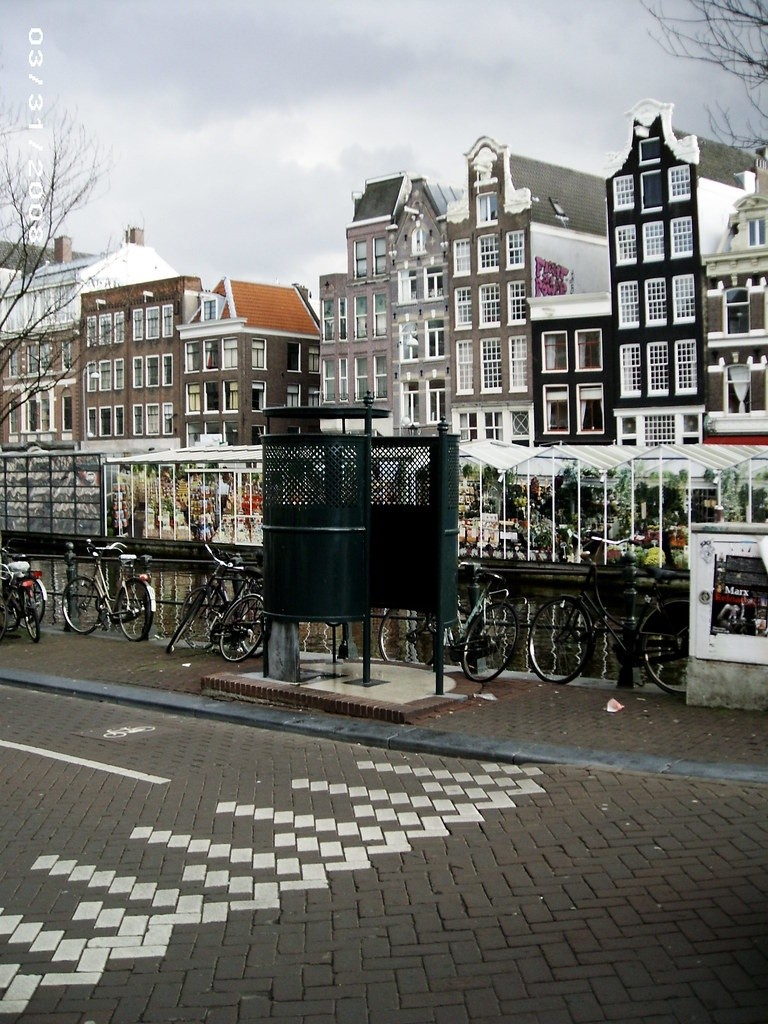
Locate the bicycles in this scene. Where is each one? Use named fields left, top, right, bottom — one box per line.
left=525, top=529, right=690, bottom=695
left=61, top=539, right=157, bottom=642
left=377, top=566, right=520, bottom=683
left=165, top=544, right=267, bottom=663
left=0, top=538, right=48, bottom=644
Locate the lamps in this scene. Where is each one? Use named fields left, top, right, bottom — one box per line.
left=143, top=290, right=154, bottom=303
left=96, top=299, right=106, bottom=310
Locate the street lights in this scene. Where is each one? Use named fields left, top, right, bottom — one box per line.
left=396, top=323, right=418, bottom=436
